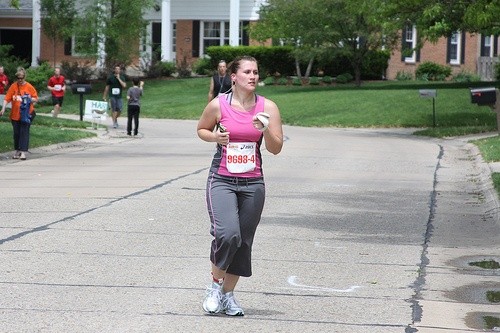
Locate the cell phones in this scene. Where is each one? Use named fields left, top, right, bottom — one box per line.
left=220, top=126, right=226, bottom=133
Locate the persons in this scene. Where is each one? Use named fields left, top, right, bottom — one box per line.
left=0, top=67, right=38, bottom=160
left=197, top=55, right=283, bottom=316
left=102, top=65, right=126, bottom=128
left=47, top=68, right=66, bottom=118
left=0, top=65, right=9, bottom=94
left=126, top=78, right=144, bottom=136
left=207, top=60, right=232, bottom=103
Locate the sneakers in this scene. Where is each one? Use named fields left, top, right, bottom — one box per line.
left=203, top=281, right=223, bottom=313
left=220, top=291, right=244, bottom=315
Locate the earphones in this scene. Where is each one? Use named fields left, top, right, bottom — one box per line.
left=233, top=77, right=235, bottom=81
